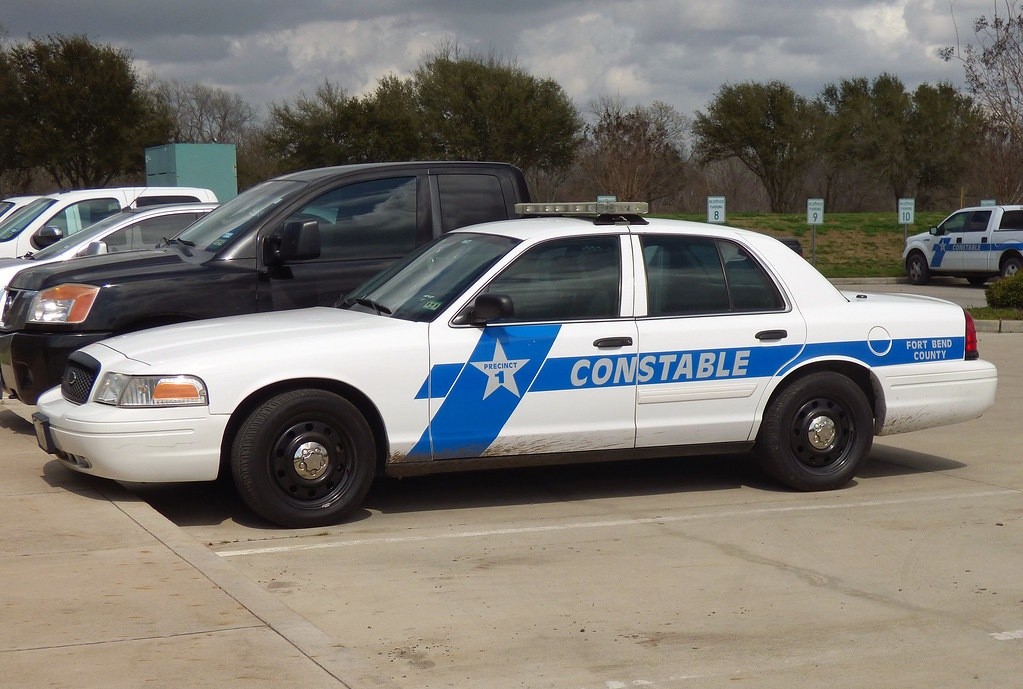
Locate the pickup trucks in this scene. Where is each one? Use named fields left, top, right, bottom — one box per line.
left=1, top=161, right=804, bottom=411
left=903, top=204, right=1022, bottom=286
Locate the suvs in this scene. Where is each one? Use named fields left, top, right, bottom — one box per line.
left=1, top=186, right=217, bottom=324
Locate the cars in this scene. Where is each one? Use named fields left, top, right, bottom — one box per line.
left=31, top=199, right=999, bottom=528
